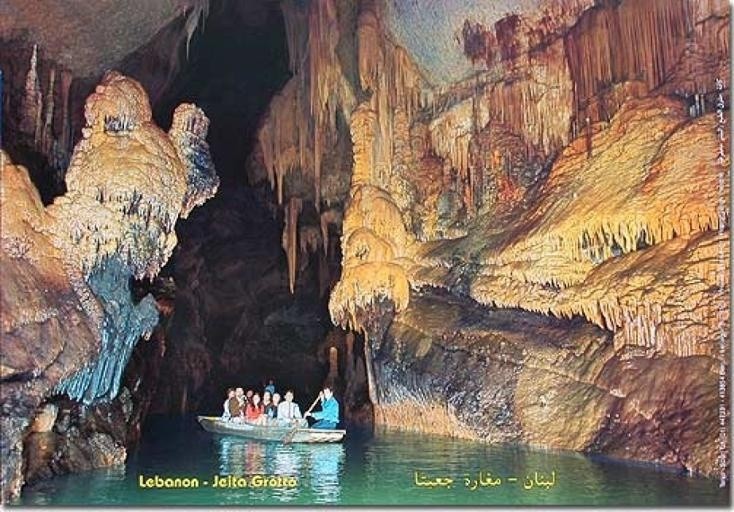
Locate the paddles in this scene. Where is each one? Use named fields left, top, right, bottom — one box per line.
left=283, top=394, right=320, bottom=445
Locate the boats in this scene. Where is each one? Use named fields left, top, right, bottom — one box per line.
left=197, top=415, right=346, bottom=443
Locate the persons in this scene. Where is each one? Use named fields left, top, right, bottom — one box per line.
left=222, top=375, right=309, bottom=428
left=303, top=383, right=340, bottom=428
